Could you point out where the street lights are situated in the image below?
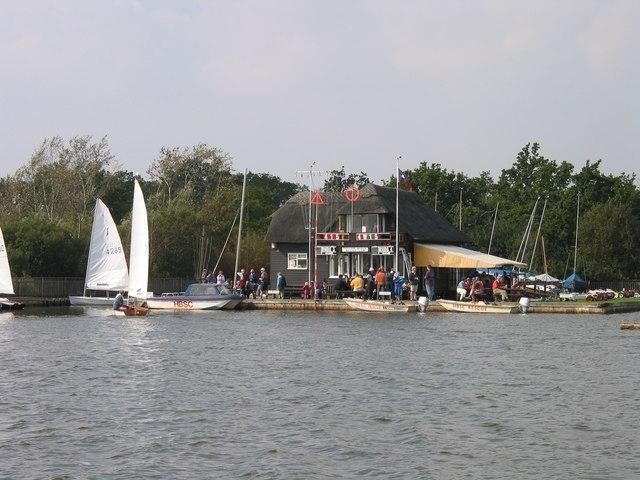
[394,155,402,277]
[294,161,331,298]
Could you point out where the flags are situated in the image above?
[398,170,413,193]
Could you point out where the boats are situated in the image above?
[132,282,244,311]
[437,295,530,314]
[342,296,429,313]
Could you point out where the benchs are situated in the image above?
[336,290,358,300]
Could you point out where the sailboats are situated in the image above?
[113,178,151,316]
[69,198,153,308]
[0,226,25,312]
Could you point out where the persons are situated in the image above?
[408,265,420,301]
[456,270,521,302]
[276,271,287,300]
[332,265,406,301]
[200,267,271,300]
[423,264,437,301]
